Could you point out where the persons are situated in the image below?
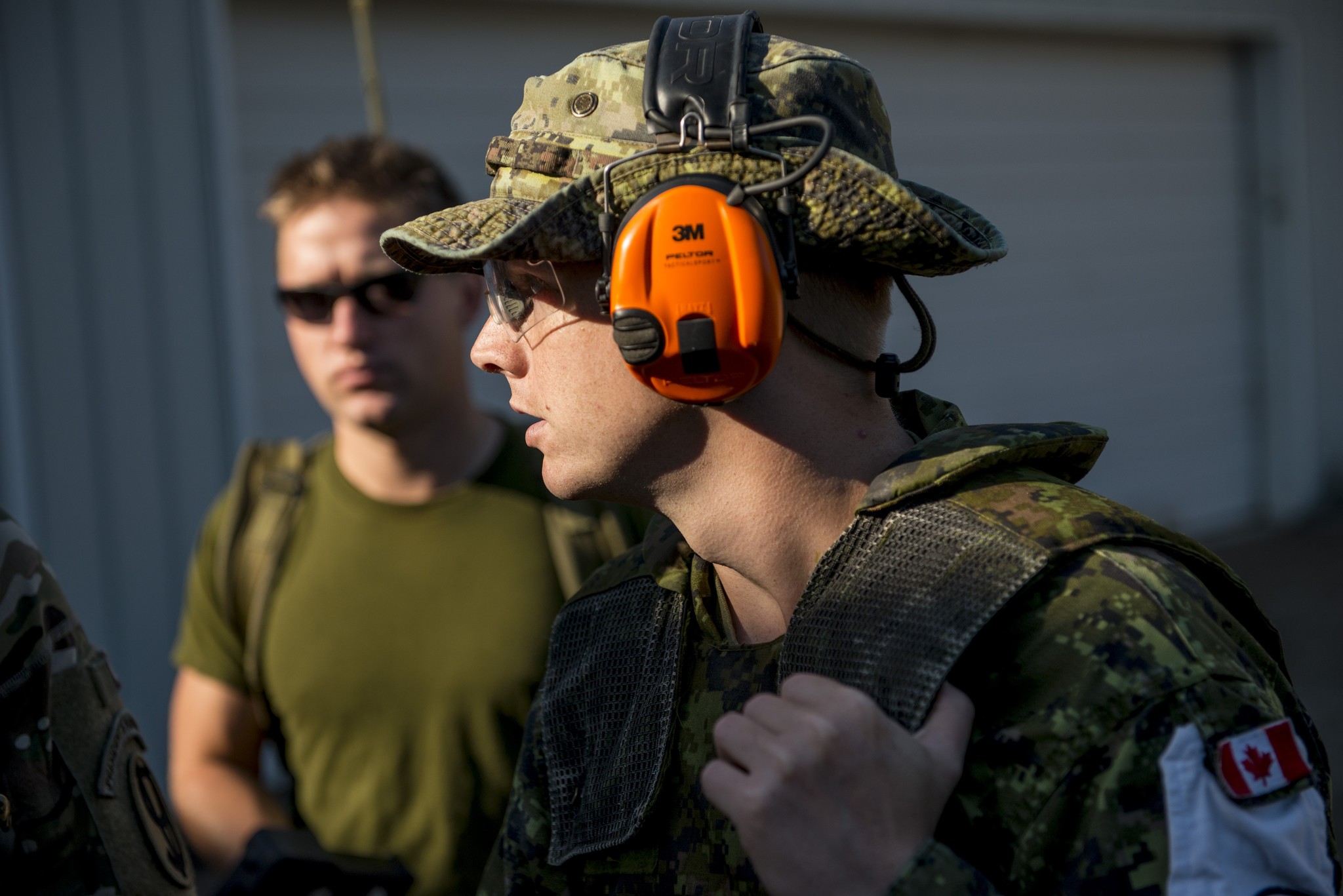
[0,504,205,895]
[159,132,636,896]
[375,16,1343,895]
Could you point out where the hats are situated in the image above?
[381,31,1007,278]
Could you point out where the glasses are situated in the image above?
[482,257,544,333]
[276,268,426,326]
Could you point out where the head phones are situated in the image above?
[598,9,784,411]
